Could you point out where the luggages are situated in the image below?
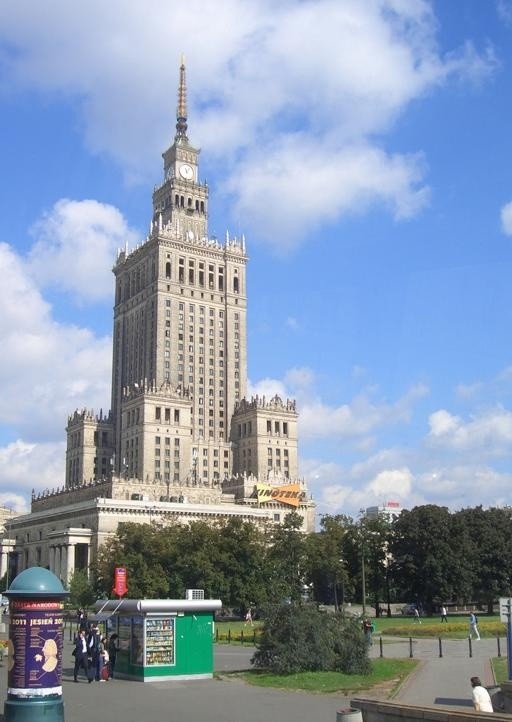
[101,664,109,681]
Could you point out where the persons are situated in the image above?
[470,675,494,713]
[413,608,422,624]
[360,617,376,649]
[243,608,255,626]
[440,605,448,624]
[70,607,121,684]
[466,611,481,641]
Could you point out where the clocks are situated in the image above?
[165,164,193,180]
